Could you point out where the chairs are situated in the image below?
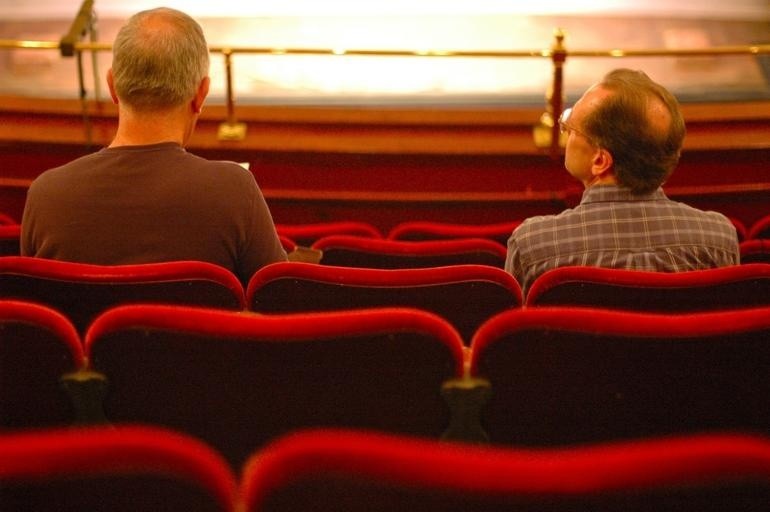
[467,304,769,424]
[2,422,236,512]
[246,261,524,345]
[524,261,770,306]
[1,254,246,339]
[237,424,769,512]
[2,177,748,261]
[86,302,467,424]
[0,299,85,421]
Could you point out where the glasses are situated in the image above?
[558,107,599,147]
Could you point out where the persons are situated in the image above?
[19,6,290,290]
[504,66,741,304]
